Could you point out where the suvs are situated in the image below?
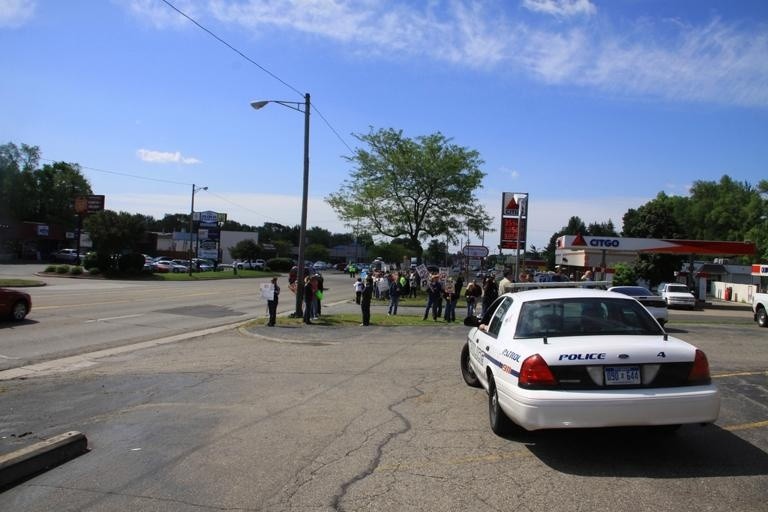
[236,258,265,270]
[695,262,731,276]
[659,283,696,309]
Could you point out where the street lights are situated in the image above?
[189,182,211,278]
[510,193,530,285]
[248,89,309,316]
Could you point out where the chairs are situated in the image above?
[531,306,627,330]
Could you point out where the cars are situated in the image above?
[0,286,33,323]
[137,254,217,273]
[657,281,666,296]
[456,286,721,441]
[305,260,370,272]
[598,284,667,328]
[452,265,498,278]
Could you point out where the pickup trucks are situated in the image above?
[750,291,768,328]
[55,247,86,258]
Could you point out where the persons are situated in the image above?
[289,260,323,325]
[267,276,280,327]
[233,259,237,275]
[346,256,596,326]
